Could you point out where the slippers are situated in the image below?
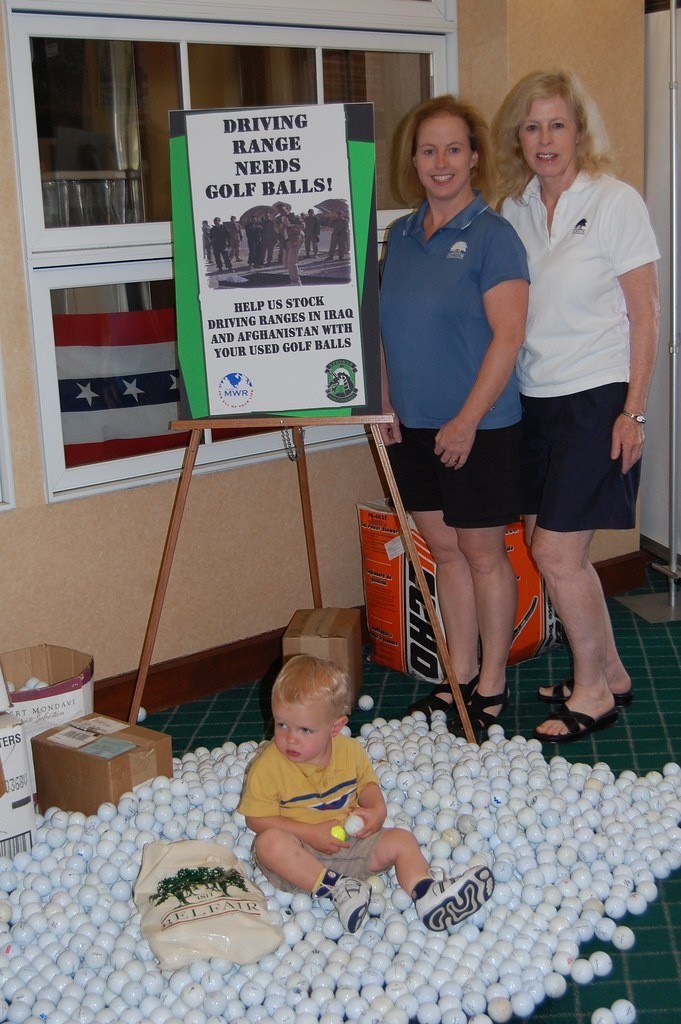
[532,698,619,743]
[538,676,635,708]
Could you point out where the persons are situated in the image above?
[377,95,534,746]
[490,69,661,744]
[199,199,350,287]
[235,658,497,934]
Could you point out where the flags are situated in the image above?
[54,308,295,470]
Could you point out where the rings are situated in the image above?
[448,458,458,464]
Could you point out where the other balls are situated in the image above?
[1,692,681,1023]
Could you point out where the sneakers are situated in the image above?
[416,866,495,930]
[313,872,371,934]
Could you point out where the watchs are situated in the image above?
[622,410,646,424]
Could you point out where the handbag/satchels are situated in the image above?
[132,839,284,969]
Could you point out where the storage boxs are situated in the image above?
[0,643,173,859]
[282,607,365,705]
[357,497,566,683]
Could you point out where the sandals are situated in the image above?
[407,673,481,724]
[450,679,511,734]
